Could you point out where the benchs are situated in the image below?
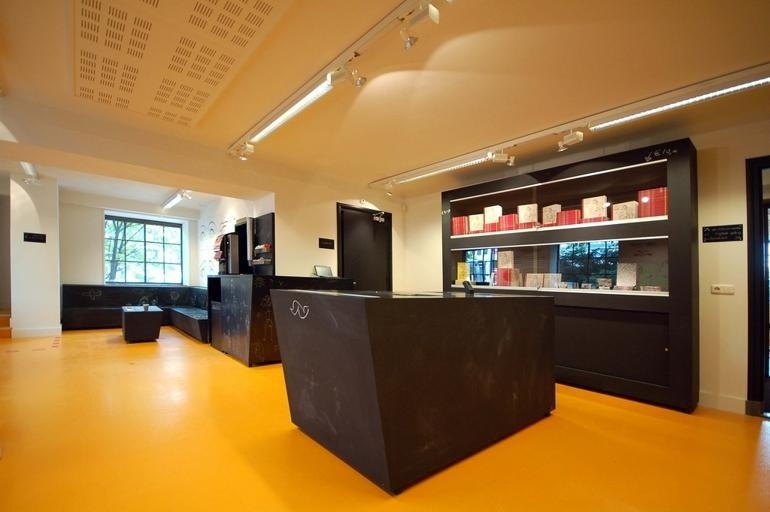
[62,284,211,346]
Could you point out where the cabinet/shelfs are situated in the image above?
[439,152,701,415]
